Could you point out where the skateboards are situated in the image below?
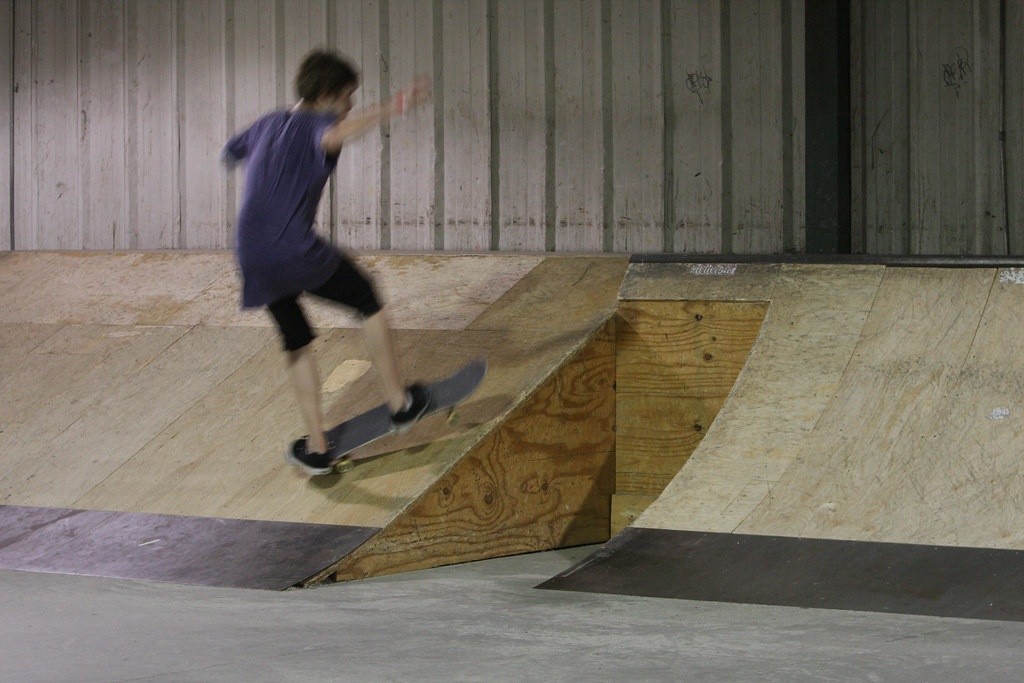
[292,355,488,473]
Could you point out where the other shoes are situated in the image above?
[390,382,432,434]
[291,436,332,474]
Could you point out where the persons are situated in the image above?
[222,49,432,475]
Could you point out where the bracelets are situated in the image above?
[395,92,403,113]
